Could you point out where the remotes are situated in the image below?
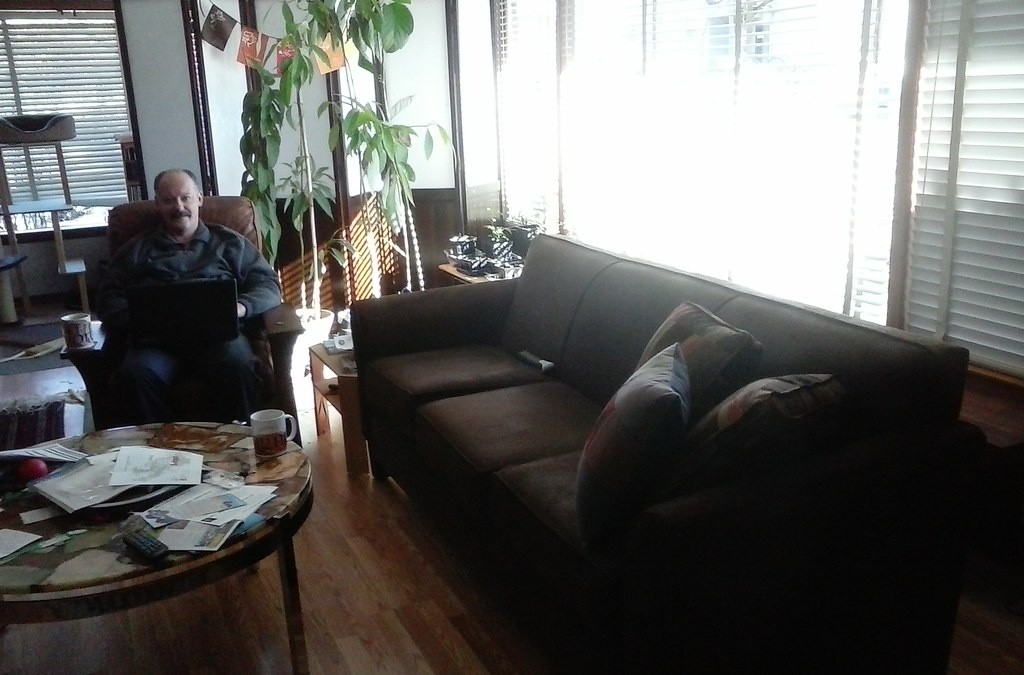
[123,529,167,560]
[517,350,555,373]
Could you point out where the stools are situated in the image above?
[0,255,29,326]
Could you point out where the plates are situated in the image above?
[90,446,173,509]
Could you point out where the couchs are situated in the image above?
[349,233,973,675]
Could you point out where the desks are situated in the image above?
[0,205,113,247]
[308,341,371,476]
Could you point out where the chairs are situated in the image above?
[58,192,305,457]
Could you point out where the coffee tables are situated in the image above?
[0,422,315,675]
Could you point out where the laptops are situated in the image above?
[128,278,239,345]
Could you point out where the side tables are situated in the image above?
[436,258,490,286]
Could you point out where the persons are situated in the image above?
[95,168,284,425]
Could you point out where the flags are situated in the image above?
[314,34,345,75]
[237,26,269,70]
[277,38,295,75]
[201,5,237,51]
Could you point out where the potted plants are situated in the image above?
[240,1,456,352]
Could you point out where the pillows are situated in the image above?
[689,370,849,491]
[573,336,693,517]
[624,297,767,424]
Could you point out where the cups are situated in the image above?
[250,409,297,458]
[61,313,95,350]
